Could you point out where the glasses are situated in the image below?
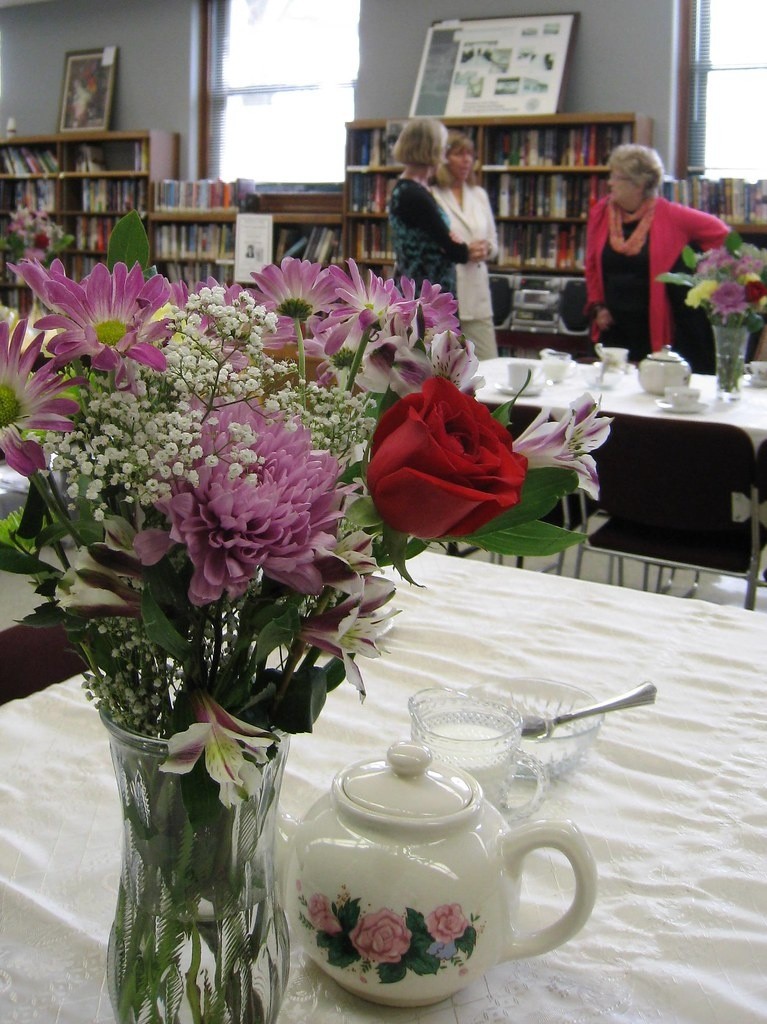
[608,172,634,183]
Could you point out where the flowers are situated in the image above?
[655,231,767,330]
[0,208,73,269]
[0,210,614,778]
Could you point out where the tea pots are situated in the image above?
[638,344,692,394]
[287,742,597,1008]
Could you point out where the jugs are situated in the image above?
[539,348,576,383]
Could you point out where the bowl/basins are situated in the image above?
[466,677,604,780]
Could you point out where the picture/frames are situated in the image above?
[57,47,119,135]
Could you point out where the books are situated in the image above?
[349,220,400,260]
[486,173,611,219]
[348,174,399,214]
[156,224,232,260]
[0,142,147,319]
[274,227,344,264]
[485,123,634,164]
[490,221,591,268]
[162,264,235,296]
[652,176,767,225]
[350,127,476,164]
[152,178,255,211]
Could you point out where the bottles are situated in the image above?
[593,342,628,389]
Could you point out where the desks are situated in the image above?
[470,359,767,453]
[0,550,767,1024]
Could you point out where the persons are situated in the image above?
[388,116,499,361]
[584,144,731,375]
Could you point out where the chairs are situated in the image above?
[447,356,598,576]
[573,410,767,612]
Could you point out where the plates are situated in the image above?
[749,378,767,387]
[656,400,708,412]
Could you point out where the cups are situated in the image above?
[663,386,700,402]
[743,359,767,379]
[506,359,540,390]
[409,686,550,822]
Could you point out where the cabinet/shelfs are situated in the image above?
[0,112,767,342]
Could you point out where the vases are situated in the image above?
[100,712,289,1024]
[711,325,749,400]
